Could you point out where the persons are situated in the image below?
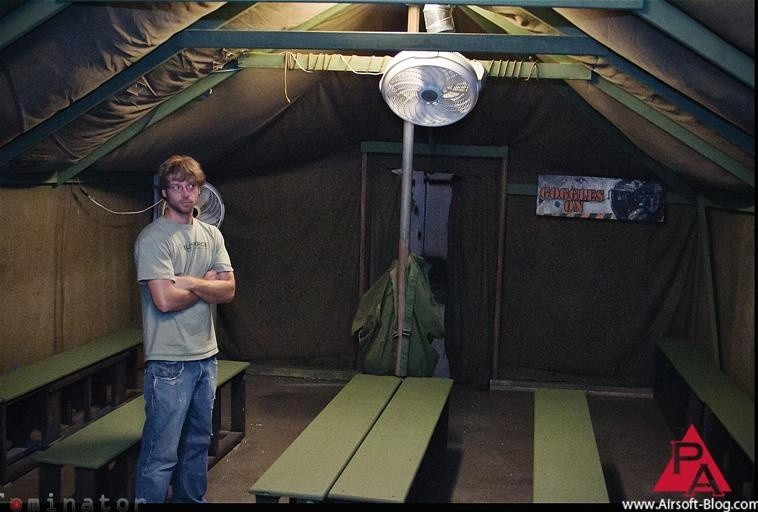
[133,155,235,512]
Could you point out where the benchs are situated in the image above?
[657,334,755,465]
[533,386,611,503]
[1,326,146,483]
[249,373,401,505]
[32,360,250,507]
[326,377,454,504]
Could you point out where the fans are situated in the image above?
[377,51,484,128]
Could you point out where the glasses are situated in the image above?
[165,182,199,192]
